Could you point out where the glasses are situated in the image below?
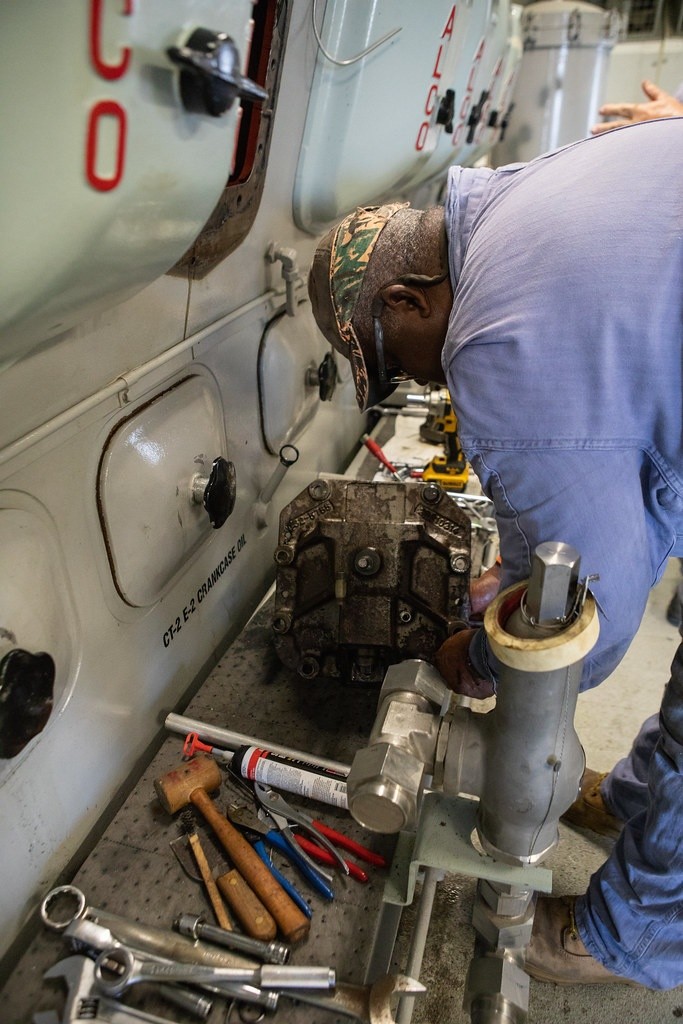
[371,273,446,385]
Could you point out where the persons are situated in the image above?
[588,79,683,135]
[305,116,683,993]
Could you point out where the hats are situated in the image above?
[307,199,410,414]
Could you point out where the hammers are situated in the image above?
[152,753,311,945]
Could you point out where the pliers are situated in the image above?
[255,782,385,883]
[226,802,336,920]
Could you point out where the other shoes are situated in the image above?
[525,893,652,991]
[560,765,626,839]
